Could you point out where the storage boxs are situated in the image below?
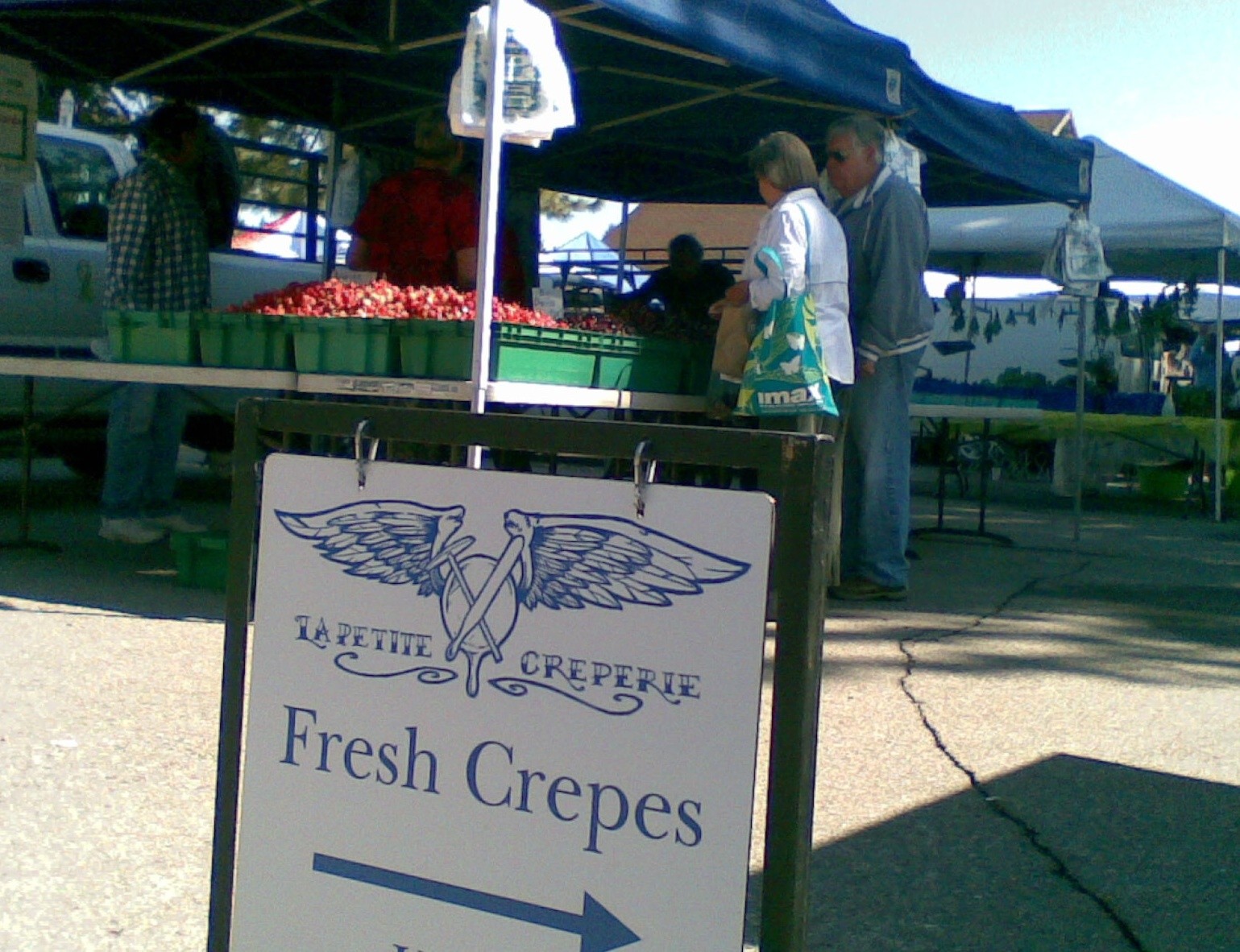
[108,311,691,590]
[912,378,1173,418]
[0,56,39,188]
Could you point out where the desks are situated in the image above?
[908,405,1240,548]
[295,373,713,495]
[0,352,300,553]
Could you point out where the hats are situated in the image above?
[149,102,214,138]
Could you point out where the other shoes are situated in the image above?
[828,576,910,600]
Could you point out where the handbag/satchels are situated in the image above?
[712,203,840,419]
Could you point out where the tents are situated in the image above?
[0,0,1240,551]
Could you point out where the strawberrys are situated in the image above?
[213,278,640,334]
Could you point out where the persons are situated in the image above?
[345,107,525,463]
[98,105,214,546]
[1189,324,1230,490]
[708,131,855,587]
[617,234,735,323]
[131,99,243,250]
[827,114,935,603]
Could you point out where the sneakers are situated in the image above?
[96,517,166,545]
[144,514,209,533]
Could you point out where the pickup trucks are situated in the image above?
[0,122,385,486]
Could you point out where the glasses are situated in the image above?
[824,147,865,163]
